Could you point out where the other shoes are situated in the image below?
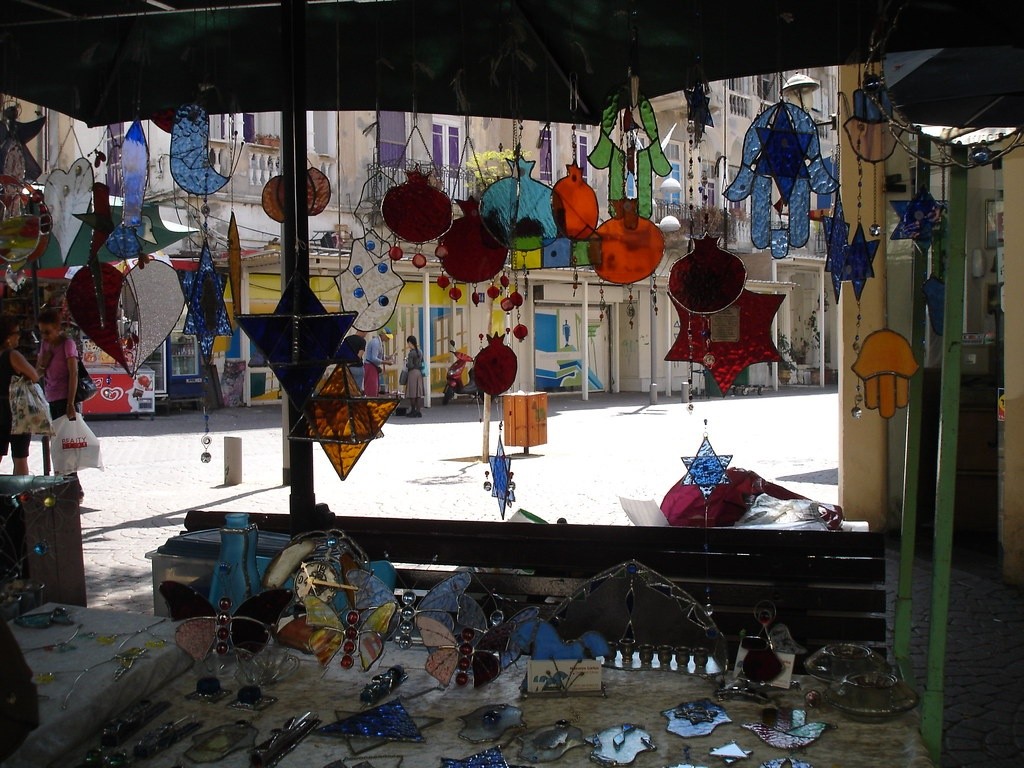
[407,410,423,418]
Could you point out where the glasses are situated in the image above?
[39,329,57,335]
[11,330,23,337]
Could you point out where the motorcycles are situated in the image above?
[442,339,496,405]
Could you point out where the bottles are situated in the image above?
[171,333,195,375]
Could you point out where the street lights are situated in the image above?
[649,174,681,405]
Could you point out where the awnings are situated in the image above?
[0,0,1024,128]
[219,248,800,292]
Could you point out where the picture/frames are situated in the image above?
[985,199,1004,249]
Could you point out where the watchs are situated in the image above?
[67,403,74,407]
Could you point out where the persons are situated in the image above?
[363,327,394,397]
[340,330,366,388]
[35,309,85,504]
[405,336,425,418]
[0,316,40,475]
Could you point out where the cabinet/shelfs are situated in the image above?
[954,406,998,532]
[0,472,87,608]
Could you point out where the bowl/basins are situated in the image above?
[803,644,891,686]
[823,671,919,722]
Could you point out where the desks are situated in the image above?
[5,602,934,768]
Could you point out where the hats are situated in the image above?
[381,327,393,339]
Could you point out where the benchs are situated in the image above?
[158,509,888,657]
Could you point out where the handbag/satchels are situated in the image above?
[377,367,382,374]
[8,375,57,436]
[68,360,97,405]
[51,412,105,474]
[398,370,408,385]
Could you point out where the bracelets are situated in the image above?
[39,364,45,369]
